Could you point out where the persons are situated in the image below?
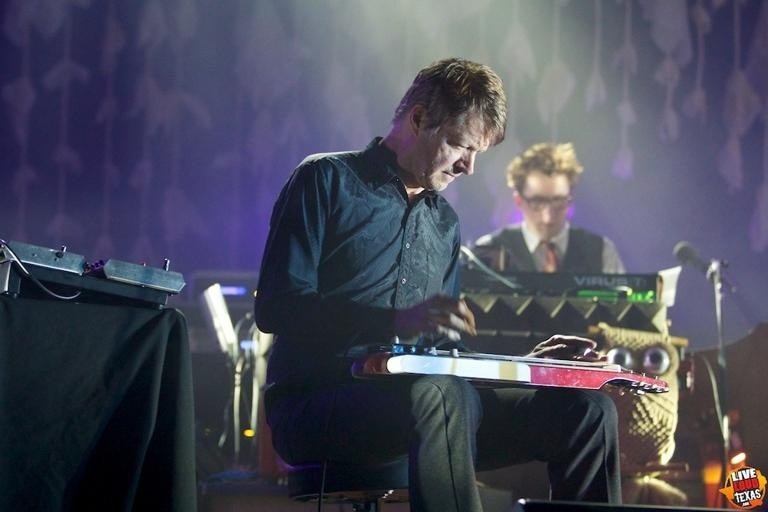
[467,138,629,320]
[255,58,670,510]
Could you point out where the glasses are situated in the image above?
[520,190,577,212]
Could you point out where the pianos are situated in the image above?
[181,267,681,307]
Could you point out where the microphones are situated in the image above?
[672,241,737,294]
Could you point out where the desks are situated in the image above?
[1,293,196,512]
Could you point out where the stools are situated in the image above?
[286,458,408,512]
